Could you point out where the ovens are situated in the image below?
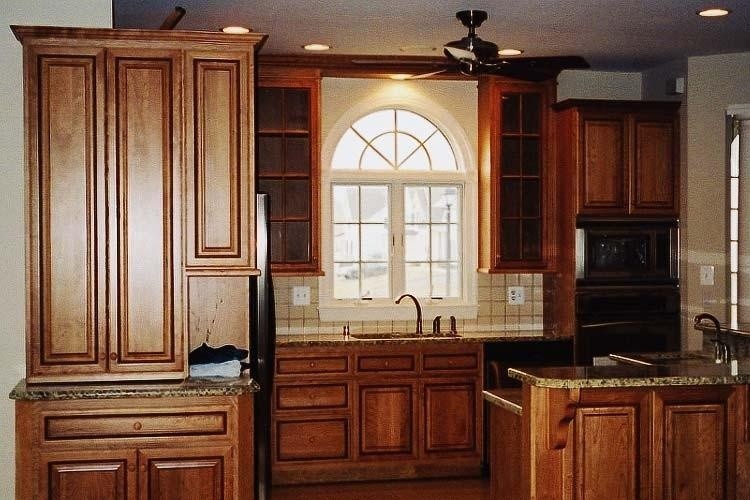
[576,291,681,366]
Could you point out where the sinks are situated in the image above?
[422,333,462,337]
[352,330,420,338]
[640,351,706,362]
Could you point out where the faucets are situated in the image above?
[394,293,423,334]
[693,313,723,366]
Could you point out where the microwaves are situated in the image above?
[577,226,674,281]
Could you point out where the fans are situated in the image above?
[408,13,589,86]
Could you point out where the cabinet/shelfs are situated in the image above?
[185,50,252,268]
[23,45,186,374]
[276,354,350,462]
[421,352,480,452]
[36,404,235,500]
[492,80,558,274]
[578,107,681,217]
[255,67,321,270]
[356,354,417,456]
